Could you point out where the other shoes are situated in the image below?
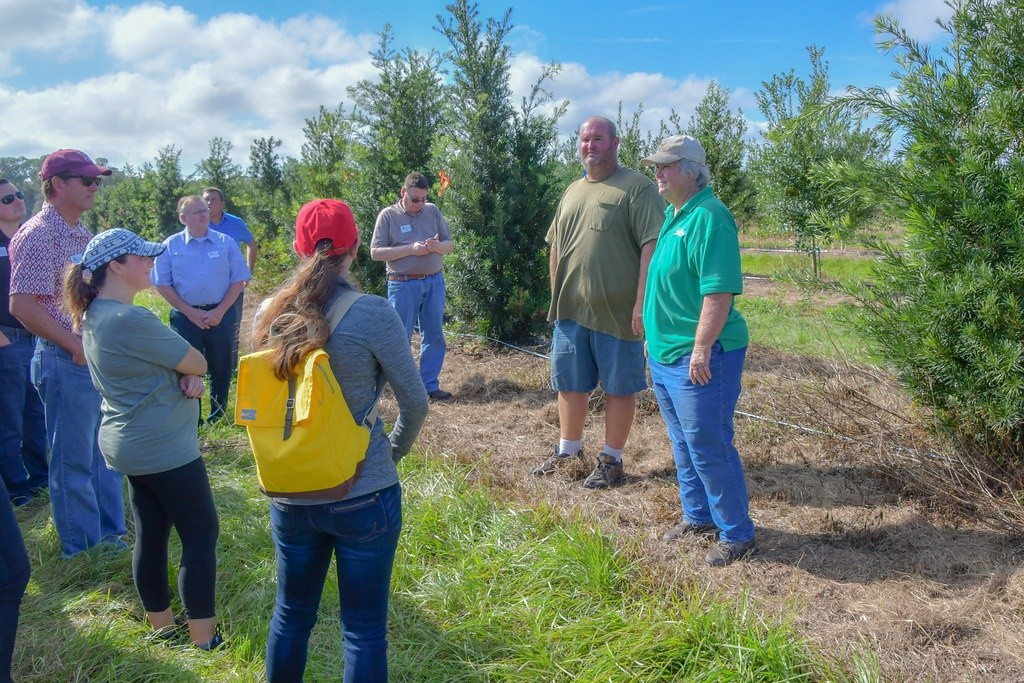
[206,622,228,651]
[426,389,453,400]
[9,492,36,511]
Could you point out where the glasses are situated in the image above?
[0,191,25,206]
[62,174,102,187]
[183,207,211,219]
[404,189,427,203]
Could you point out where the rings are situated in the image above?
[201,382,205,388]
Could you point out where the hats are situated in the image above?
[296,200,359,260]
[639,135,707,170]
[41,148,112,182]
[81,227,167,272]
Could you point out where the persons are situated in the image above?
[641,135,759,565]
[7,149,130,558]
[232,198,429,683]
[0,179,49,505]
[63,228,232,652]
[150,195,253,426]
[371,172,453,401]
[201,187,258,383]
[0,477,32,682]
[531,115,668,489]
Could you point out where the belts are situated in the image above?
[388,273,432,282]
[193,304,217,312]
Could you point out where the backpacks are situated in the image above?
[234,291,391,499]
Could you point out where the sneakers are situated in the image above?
[529,443,585,477]
[661,520,717,545]
[705,536,756,566]
[583,453,624,489]
